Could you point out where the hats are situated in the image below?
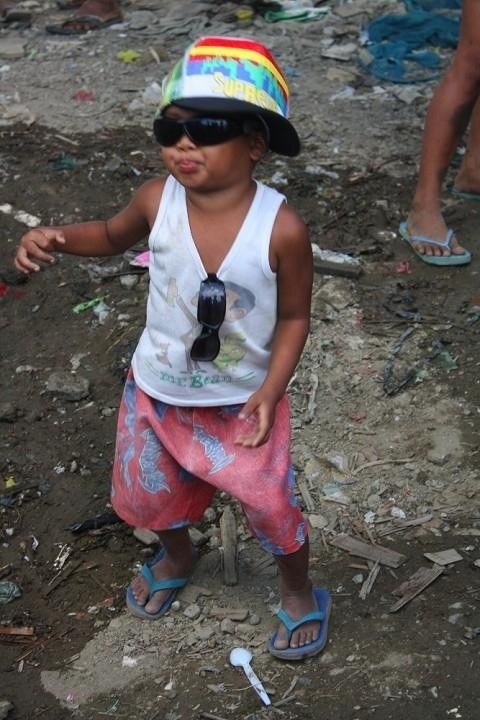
[155,35,300,156]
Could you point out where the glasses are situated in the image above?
[190,273,226,362]
[154,114,246,146]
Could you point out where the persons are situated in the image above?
[46,0,123,35]
[13,35,334,660]
[398,0,480,266]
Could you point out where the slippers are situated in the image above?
[399,222,471,266]
[125,545,191,621]
[267,586,332,660]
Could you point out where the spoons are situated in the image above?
[229,646,272,707]
